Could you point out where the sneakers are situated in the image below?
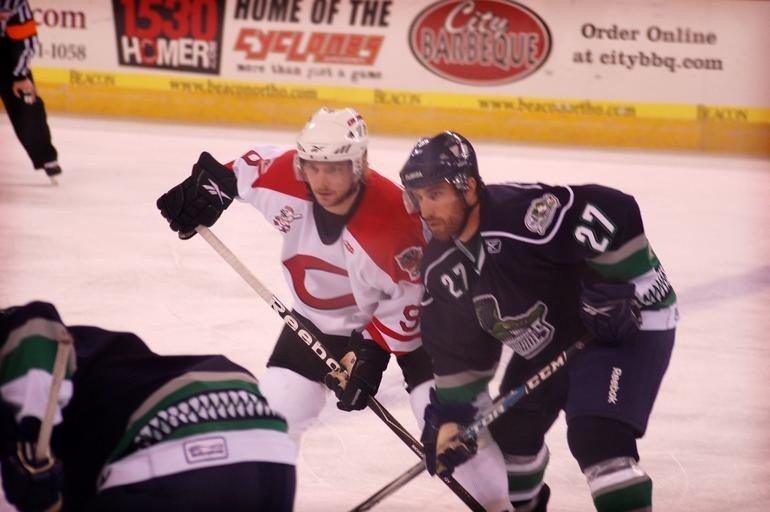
[43,162,61,175]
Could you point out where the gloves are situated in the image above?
[324,329,389,410]
[579,279,644,345]
[156,152,237,240]
[0,441,67,512]
[421,387,478,477]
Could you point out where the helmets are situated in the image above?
[399,130,479,211]
[294,108,369,181]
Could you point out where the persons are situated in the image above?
[399,130,679,512]
[0,0,62,177]
[0,300,296,512]
[156,107,514,512]
